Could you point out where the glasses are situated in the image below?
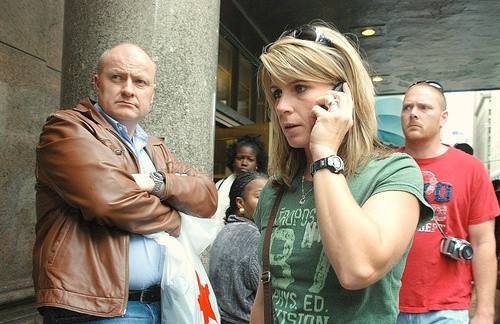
[409,79,443,90]
[278,26,335,48]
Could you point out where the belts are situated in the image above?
[127,287,160,304]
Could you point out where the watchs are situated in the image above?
[307,152,345,178]
[148,171,167,195]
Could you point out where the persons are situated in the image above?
[450,142,475,157]
[207,133,264,221]
[32,41,219,323]
[490,178,499,291]
[247,19,424,323]
[207,168,270,323]
[381,78,500,323]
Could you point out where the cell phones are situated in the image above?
[320,80,343,111]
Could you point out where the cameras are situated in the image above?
[441,236,474,260]
